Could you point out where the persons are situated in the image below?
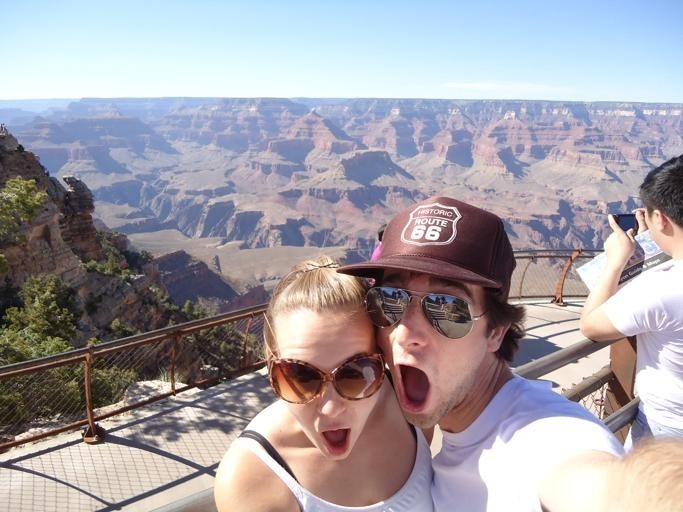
[214,257,434,512]
[604,435,683,512]
[578,155,683,458]
[367,224,386,285]
[336,194,624,512]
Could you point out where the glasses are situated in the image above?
[266,351,387,403]
[363,285,486,340]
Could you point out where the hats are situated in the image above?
[337,194,516,289]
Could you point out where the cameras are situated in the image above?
[615,213,640,235]
